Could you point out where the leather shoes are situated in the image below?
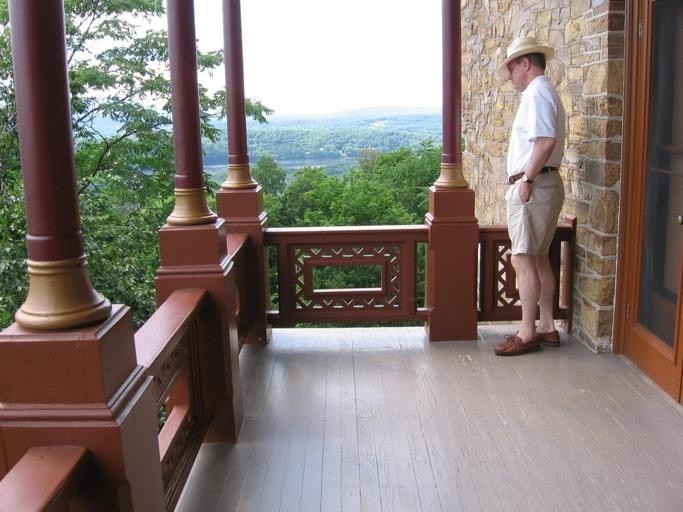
[493,333,541,357]
[535,329,561,347]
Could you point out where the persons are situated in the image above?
[495,36,566,356]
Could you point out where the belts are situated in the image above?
[508,164,558,186]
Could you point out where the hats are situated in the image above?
[496,36,556,80]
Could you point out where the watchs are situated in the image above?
[521,174,533,184]
